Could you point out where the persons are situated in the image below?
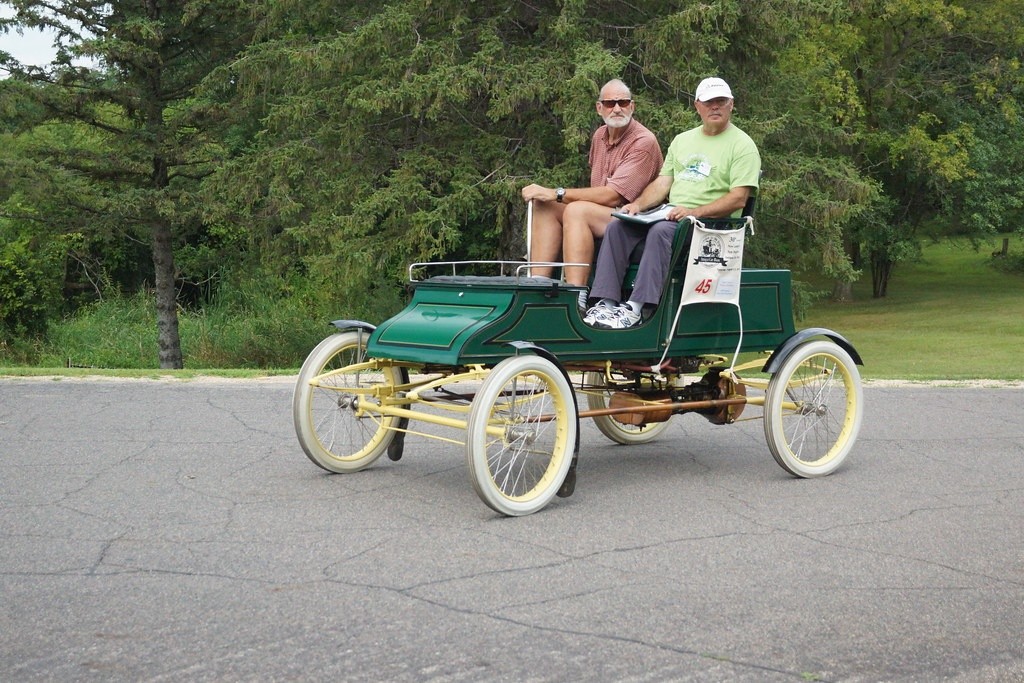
[583,76,761,332]
[522,77,664,322]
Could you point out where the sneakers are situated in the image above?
[583,298,614,327]
[593,302,643,329]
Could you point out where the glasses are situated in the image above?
[696,99,730,107]
[598,99,631,109]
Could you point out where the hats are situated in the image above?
[695,77,734,102]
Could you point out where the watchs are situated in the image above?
[556,187,566,204]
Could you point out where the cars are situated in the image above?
[293,171,866,519]
